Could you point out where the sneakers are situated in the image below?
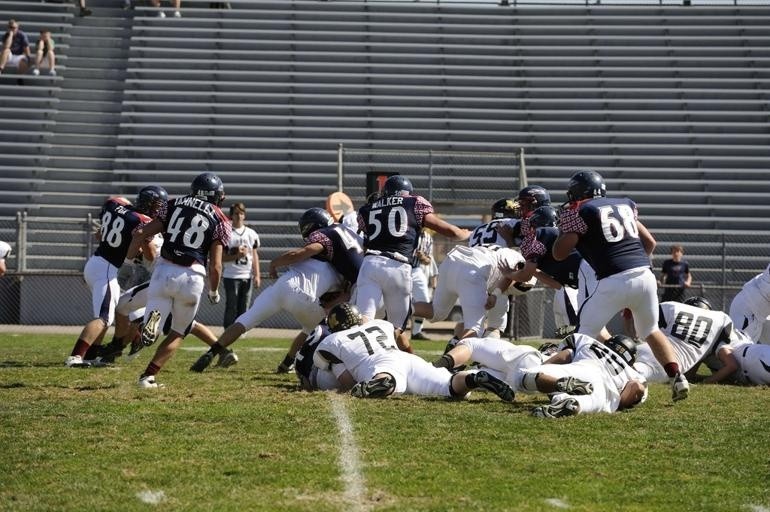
[216,351,240,368]
[533,398,580,419]
[474,371,515,401]
[190,352,214,373]
[68,341,124,368]
[128,310,162,389]
[556,377,594,396]
[672,373,690,402]
[278,363,296,373]
[351,377,396,399]
[412,332,431,341]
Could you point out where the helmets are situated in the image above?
[138,186,170,218]
[192,174,226,206]
[300,207,335,239]
[684,296,713,311]
[328,303,364,334]
[367,192,384,205]
[384,176,414,197]
[492,169,609,233]
[604,334,638,367]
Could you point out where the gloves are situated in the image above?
[209,290,221,306]
[117,258,137,292]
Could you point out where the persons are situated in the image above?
[62,168,770,422]
[32,27,57,76]
[79,0,93,18]
[0,239,12,276]
[0,18,32,86]
[152,0,182,18]
